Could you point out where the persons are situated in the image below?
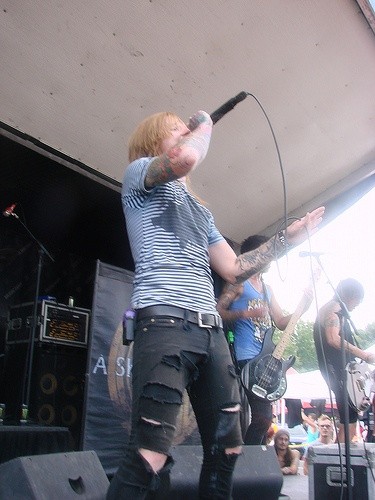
[273,429,300,475]
[216,234,314,444]
[313,278,375,444]
[300,407,319,451]
[106,109,325,500]
[302,413,336,475]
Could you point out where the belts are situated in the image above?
[137,306,223,329]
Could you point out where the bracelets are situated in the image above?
[278,231,292,249]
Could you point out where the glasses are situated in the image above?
[319,424,331,429]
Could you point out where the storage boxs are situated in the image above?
[7,297,91,350]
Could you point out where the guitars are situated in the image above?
[240,268,322,403]
[346,360,375,414]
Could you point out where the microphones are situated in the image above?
[3,200,18,216]
[210,90,248,125]
[299,250,325,257]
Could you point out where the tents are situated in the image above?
[281,369,337,427]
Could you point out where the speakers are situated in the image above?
[146,444,284,500]
[0,450,111,500]
[2,342,89,451]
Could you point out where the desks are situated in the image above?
[0,426,70,463]
